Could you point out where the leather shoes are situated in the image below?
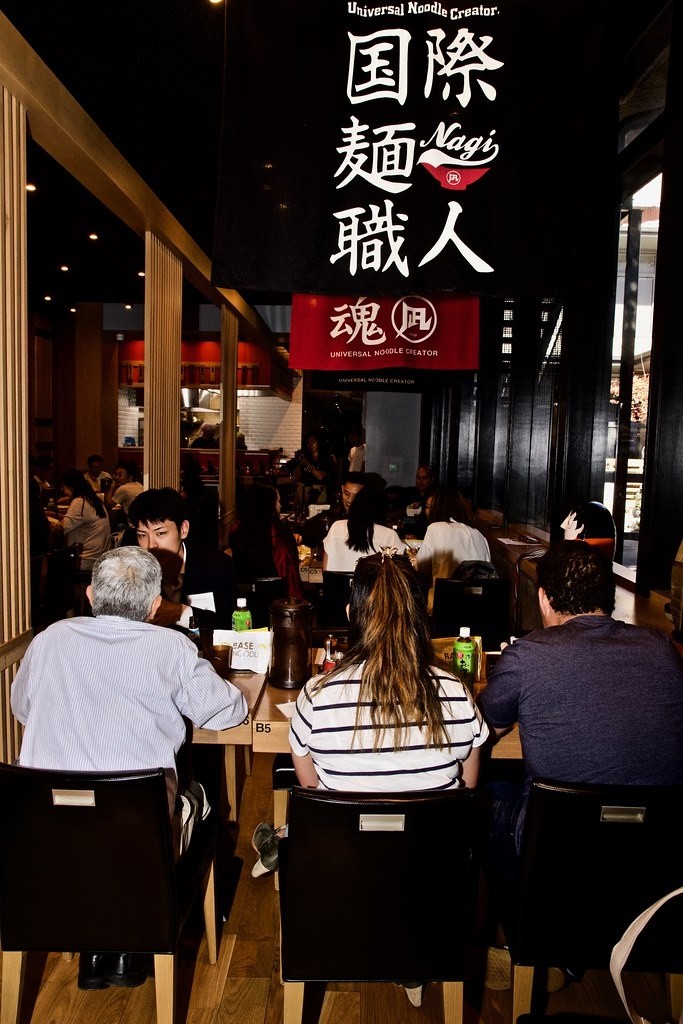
[77,952,110,990]
[103,952,149,987]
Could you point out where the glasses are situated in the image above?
[112,471,125,478]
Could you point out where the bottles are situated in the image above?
[323,633,337,660]
[232,598,253,631]
[186,616,204,658]
[453,627,476,694]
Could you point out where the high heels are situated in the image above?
[251,822,282,878]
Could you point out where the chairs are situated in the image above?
[220,571,286,634]
[431,577,513,648]
[277,792,492,1023]
[0,763,217,1024]
[505,782,682,1023]
[32,541,81,629]
[319,571,359,626]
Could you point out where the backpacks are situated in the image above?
[451,559,500,587]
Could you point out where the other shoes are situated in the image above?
[484,947,566,994]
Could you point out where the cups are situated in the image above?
[202,645,234,677]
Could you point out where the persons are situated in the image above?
[231,482,302,622]
[473,539,683,975]
[190,423,249,449]
[10,547,248,990]
[28,451,218,618]
[126,491,260,637]
[282,427,365,507]
[396,466,492,617]
[296,469,407,638]
[252,549,491,1007]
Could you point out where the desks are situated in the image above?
[41,503,550,823]
